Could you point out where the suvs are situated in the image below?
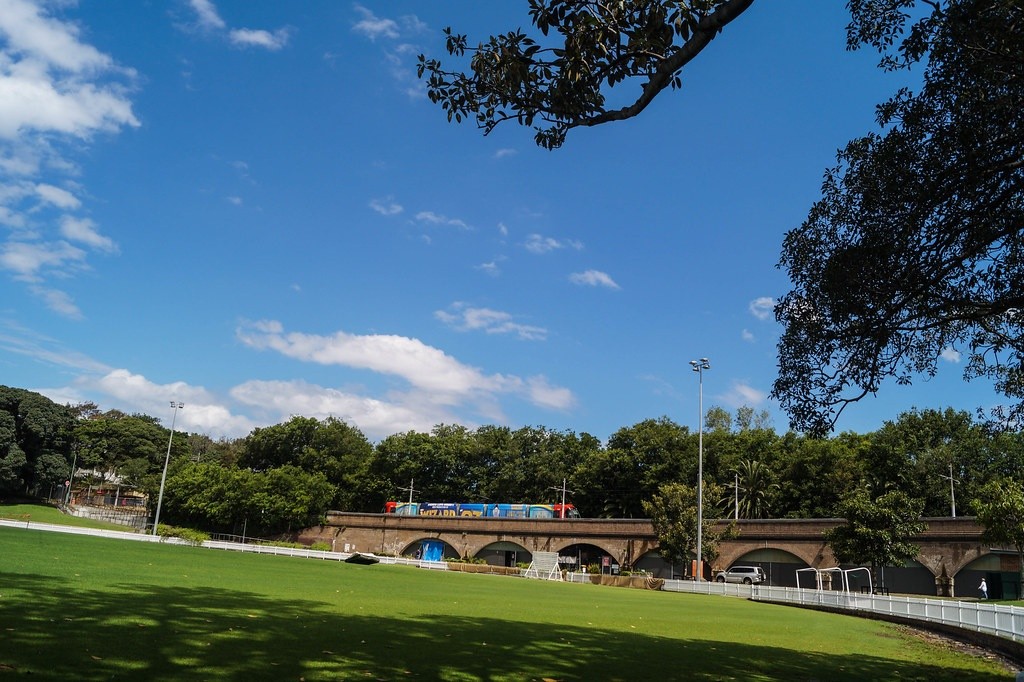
[716,566,767,585]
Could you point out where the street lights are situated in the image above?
[688,356,711,582]
[152,401,185,535]
[396,477,421,514]
[937,463,960,518]
[723,473,747,519]
[545,478,576,519]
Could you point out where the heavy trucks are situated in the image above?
[380,501,582,518]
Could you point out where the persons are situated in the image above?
[978,578,988,599]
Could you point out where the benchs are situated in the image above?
[673,575,695,580]
[861,586,889,596]
